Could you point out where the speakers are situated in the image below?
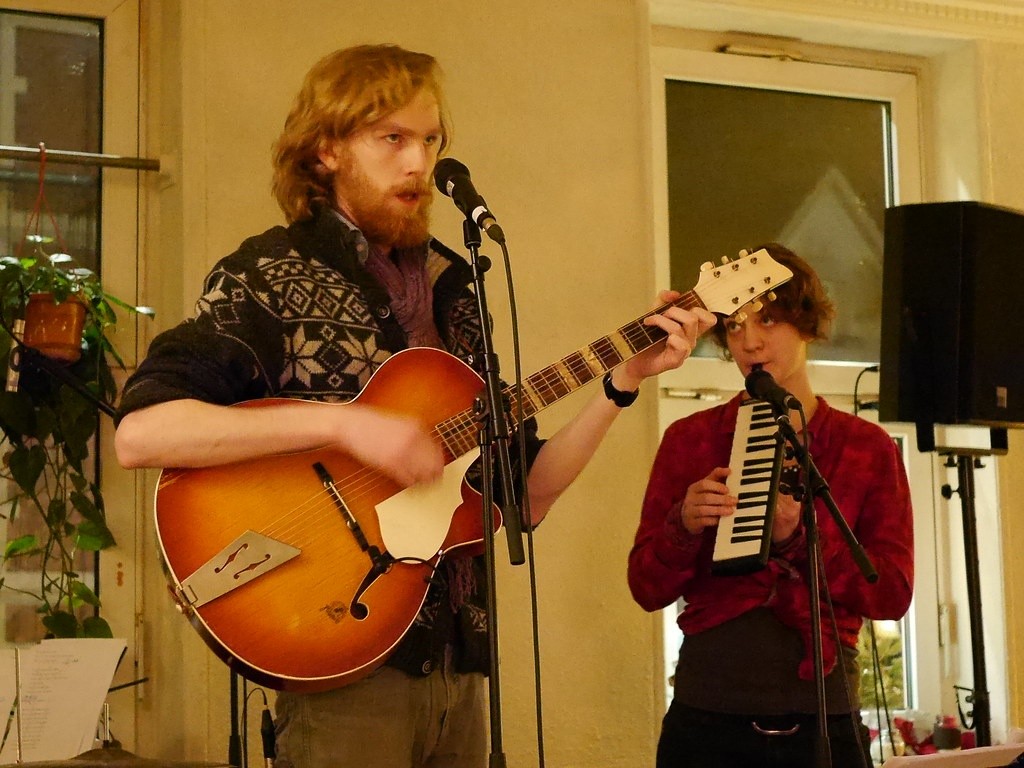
[878,201,1024,429]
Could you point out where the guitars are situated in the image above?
[154,249,792,695]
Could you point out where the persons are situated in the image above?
[114,41,716,768]
[626,243,915,768]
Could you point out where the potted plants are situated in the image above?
[0,253,157,639]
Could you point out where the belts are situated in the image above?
[746,709,805,736]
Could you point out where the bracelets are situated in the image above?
[603,371,639,407]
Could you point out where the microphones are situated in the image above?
[434,158,506,245]
[744,370,802,410]
